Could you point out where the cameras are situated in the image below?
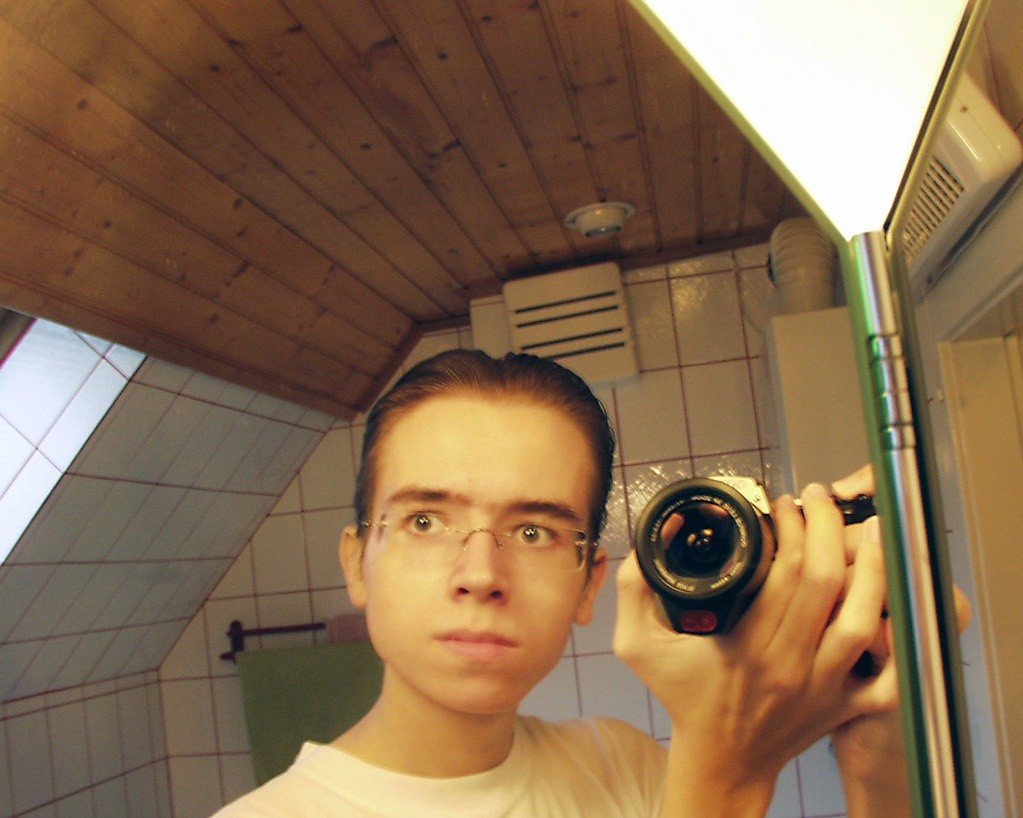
[636,474,887,638]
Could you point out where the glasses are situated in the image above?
[361,510,598,572]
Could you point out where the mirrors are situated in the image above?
[0,0,944,818]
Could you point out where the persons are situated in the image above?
[210,349,972,818]
